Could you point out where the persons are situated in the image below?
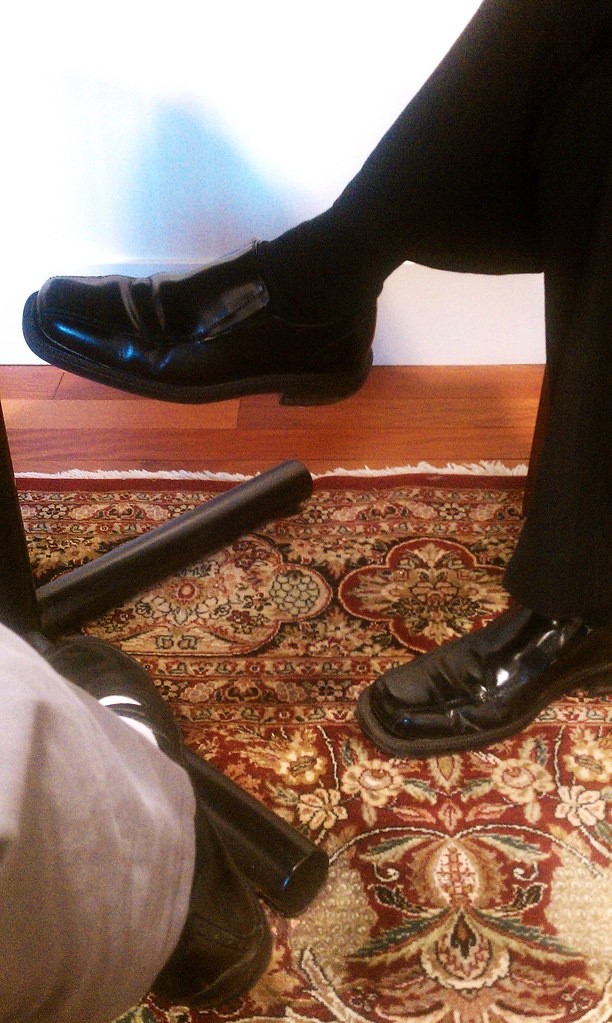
[0,623,271,1023]
[20,0,612,758]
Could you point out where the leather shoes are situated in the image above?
[40,635,272,1011]
[20,240,378,409]
[355,605,612,759]
